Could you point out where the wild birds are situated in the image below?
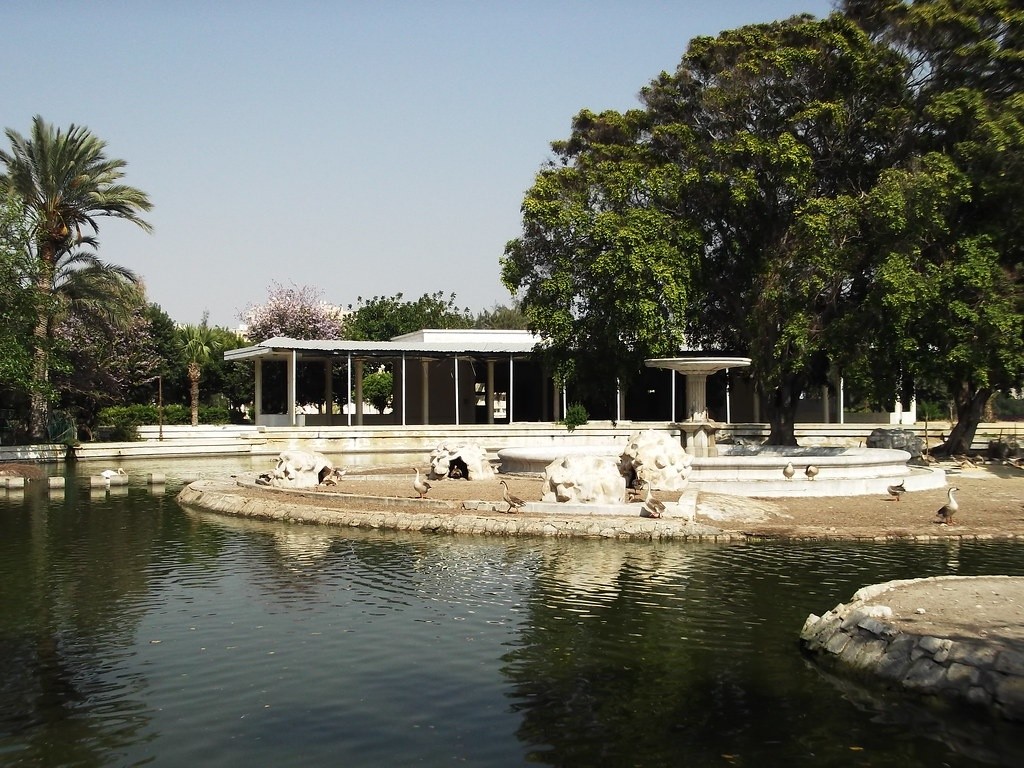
[935,487,960,525]
[888,478,907,502]
[804,464,822,480]
[641,479,667,519]
[498,480,527,514]
[100,468,127,477]
[450,464,463,478]
[413,467,432,500]
[782,461,796,480]
